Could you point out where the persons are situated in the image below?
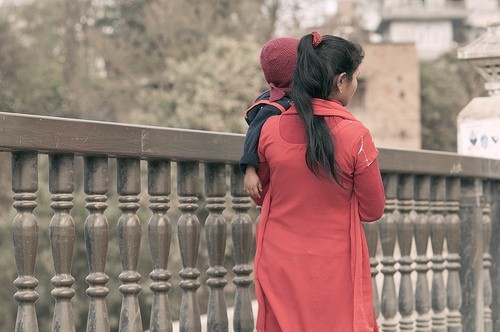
[240,37,302,198]
[253,32,386,332]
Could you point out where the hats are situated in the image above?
[260,37,300,102]
